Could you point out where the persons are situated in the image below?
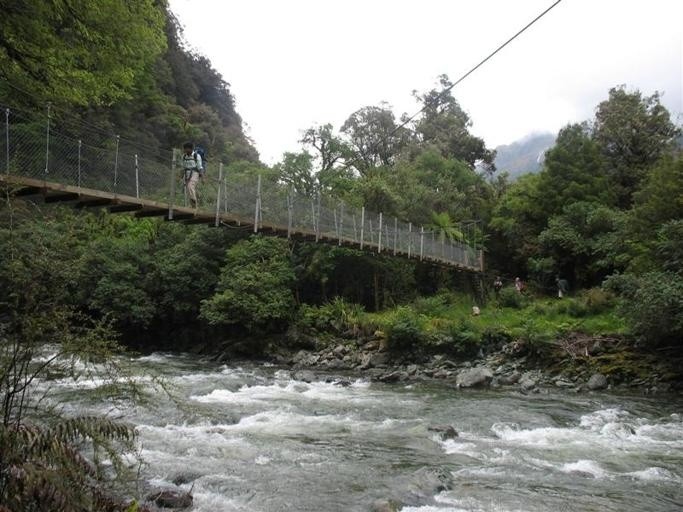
[494,277,503,299]
[179,141,205,212]
[515,277,524,294]
[554,278,566,300]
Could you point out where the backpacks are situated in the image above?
[183,147,207,177]
